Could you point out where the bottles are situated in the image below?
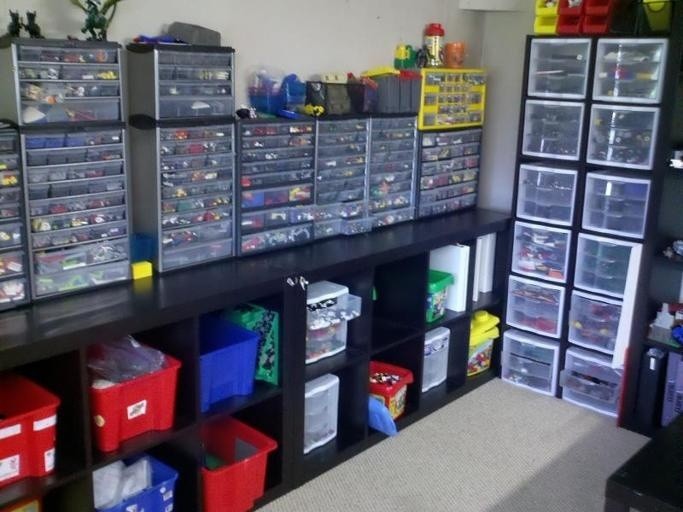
[425,24,444,68]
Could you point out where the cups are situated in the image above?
[440,41,465,68]
[393,44,424,70]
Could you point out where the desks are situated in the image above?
[604,412,683,512]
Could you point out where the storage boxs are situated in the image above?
[305,280,362,365]
[0,375,60,487]
[198,415,278,512]
[99,455,178,512]
[304,373,340,453]
[422,326,450,392]
[88,344,181,451]
[197,315,261,413]
[368,361,413,422]
[425,268,455,323]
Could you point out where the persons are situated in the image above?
[416,43,436,69]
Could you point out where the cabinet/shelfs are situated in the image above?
[618,0,683,440]
[1,21,487,311]
[243,207,514,489]
[498,36,672,419]
[0,258,299,512]
